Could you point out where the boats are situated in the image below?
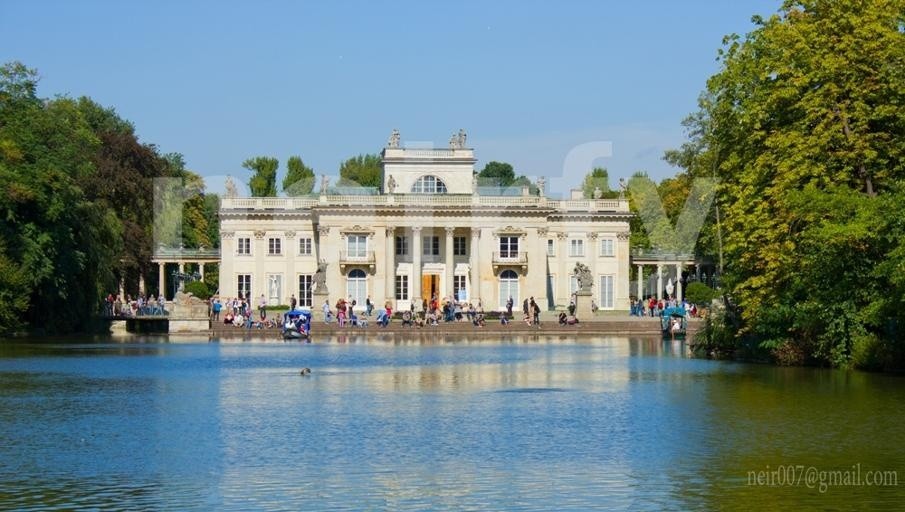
[659,307,687,339]
[282,310,312,339]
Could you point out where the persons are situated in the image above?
[536,176,544,196]
[387,175,396,193]
[449,133,457,149]
[630,295,697,341]
[387,129,400,148]
[224,173,234,198]
[205,293,597,336]
[103,293,165,318]
[593,187,603,199]
[618,177,626,199]
[312,259,329,292]
[574,261,593,291]
[321,173,329,195]
[458,128,465,148]
